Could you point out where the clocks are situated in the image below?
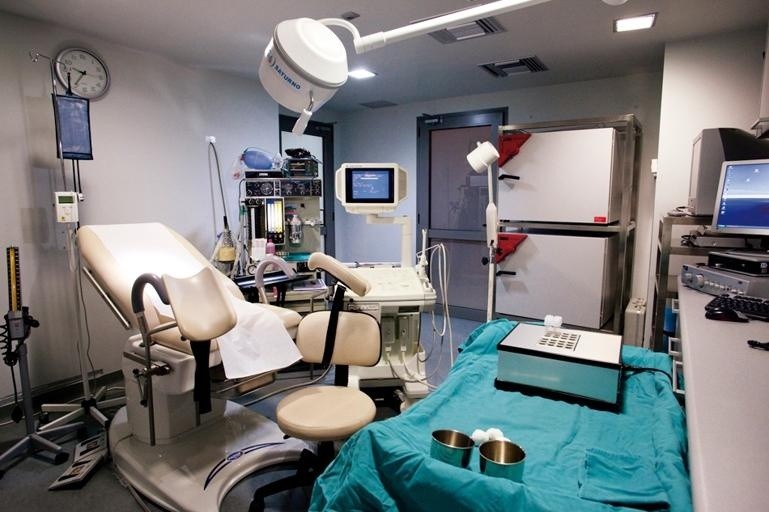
[55,47,109,100]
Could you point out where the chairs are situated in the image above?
[248,310,381,512]
[73,221,371,512]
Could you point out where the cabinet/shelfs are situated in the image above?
[493,113,643,334]
[650,215,769,352]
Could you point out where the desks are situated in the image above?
[675,275,769,511]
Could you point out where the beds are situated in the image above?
[306,318,693,512]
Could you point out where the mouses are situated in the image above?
[708,305,750,323]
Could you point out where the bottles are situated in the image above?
[290,214,303,244]
[267,239,275,271]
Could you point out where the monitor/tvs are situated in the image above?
[334,163,409,216]
[710,157,769,260]
[686,127,765,218]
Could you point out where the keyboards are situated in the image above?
[705,289,769,325]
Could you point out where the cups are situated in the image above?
[430,429,474,468]
[480,440,527,483]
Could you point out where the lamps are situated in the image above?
[258,0,546,136]
[465,140,501,323]
[208,142,237,262]
